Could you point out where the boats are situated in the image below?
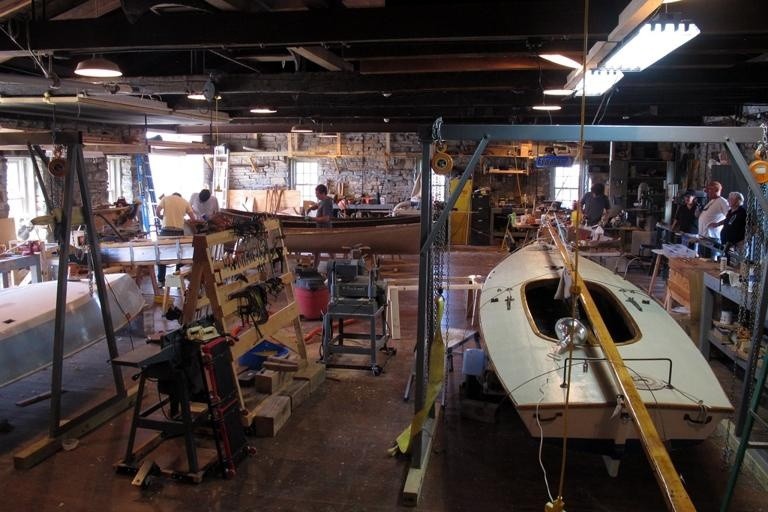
[1,274,146,389]
[476,238,734,443]
[217,207,428,256]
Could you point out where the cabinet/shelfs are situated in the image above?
[489,207,534,245]
[699,270,768,387]
[666,257,736,321]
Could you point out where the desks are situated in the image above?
[511,222,643,249]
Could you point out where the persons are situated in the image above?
[697,181,731,244]
[578,183,612,226]
[720,191,747,254]
[671,189,700,251]
[190,189,219,222]
[304,184,333,223]
[156,193,195,288]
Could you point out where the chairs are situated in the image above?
[613,245,662,280]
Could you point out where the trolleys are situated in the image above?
[313,258,396,380]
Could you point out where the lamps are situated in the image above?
[74,53,123,78]
